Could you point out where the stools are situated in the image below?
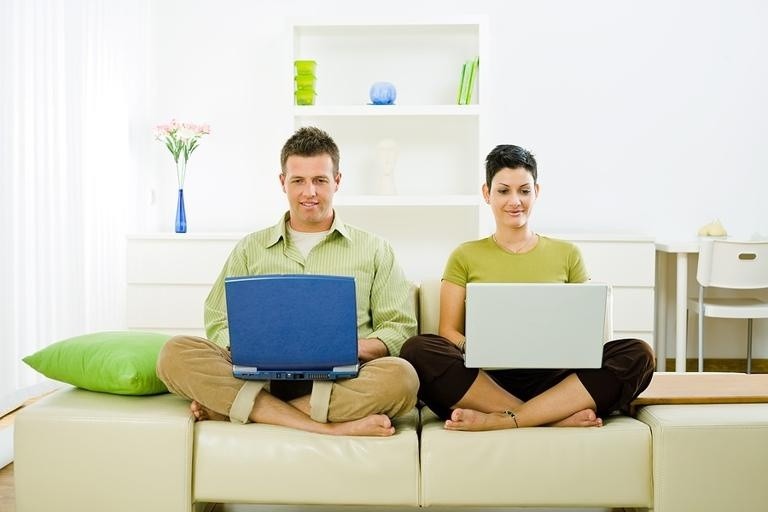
[12,388,218,512]
[634,401,768,511]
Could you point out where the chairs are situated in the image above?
[416,281,651,511]
[190,277,420,511]
[687,237,767,371]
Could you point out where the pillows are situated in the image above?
[23,328,168,397]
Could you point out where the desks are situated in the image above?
[655,231,767,372]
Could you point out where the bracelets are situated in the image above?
[454,336,467,353]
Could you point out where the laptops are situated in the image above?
[463,283,608,369]
[224,274,360,380]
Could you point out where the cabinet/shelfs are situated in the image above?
[117,227,259,343]
[554,234,657,372]
[289,20,489,240]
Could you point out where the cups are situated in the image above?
[369,83,396,106]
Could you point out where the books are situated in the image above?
[458,60,475,104]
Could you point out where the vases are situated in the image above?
[172,188,188,235]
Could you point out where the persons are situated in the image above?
[157,127,420,437]
[400,144,655,431]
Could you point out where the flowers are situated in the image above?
[152,116,211,227]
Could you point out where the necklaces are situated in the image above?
[494,231,535,254]
